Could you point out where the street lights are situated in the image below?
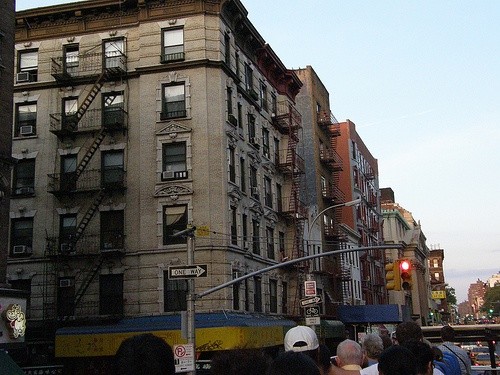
[309,198,362,363]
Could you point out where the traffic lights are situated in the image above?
[400,261,411,290]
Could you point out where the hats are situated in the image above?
[283,325,319,352]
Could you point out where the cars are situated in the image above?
[471,345,500,366]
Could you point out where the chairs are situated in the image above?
[433,351,462,375]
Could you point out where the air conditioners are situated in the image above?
[17,72,30,83]
[162,170,176,179]
[19,124,33,136]
[61,242,73,251]
[60,279,74,287]
[13,245,30,254]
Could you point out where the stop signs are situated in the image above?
[174,344,194,373]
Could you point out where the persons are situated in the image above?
[268,350,322,375]
[378,339,435,375]
[319,344,332,375]
[362,333,384,367]
[336,338,364,370]
[379,334,392,349]
[431,326,472,375]
[330,321,444,375]
[114,333,176,375]
[284,325,319,364]
